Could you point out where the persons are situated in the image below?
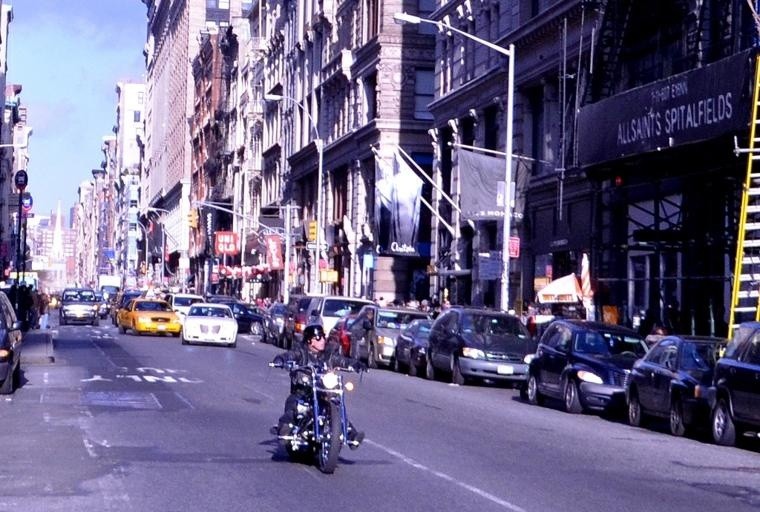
[0,279,50,351]
[508,302,587,342]
[240,295,285,316]
[360,294,457,316]
[652,326,667,336]
[271,324,369,451]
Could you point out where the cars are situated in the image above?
[623,334,730,436]
[93,285,151,328]
[180,302,241,348]
[526,319,651,414]
[203,294,268,336]
[259,295,437,378]
[0,289,24,394]
[116,298,183,338]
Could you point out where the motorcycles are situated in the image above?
[267,362,369,473]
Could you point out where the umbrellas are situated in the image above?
[581,254,594,320]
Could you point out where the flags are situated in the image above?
[457,149,529,222]
[374,156,393,256]
[135,216,343,283]
[387,151,425,256]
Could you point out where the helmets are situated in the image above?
[304,325,325,341]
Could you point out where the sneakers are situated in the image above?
[349,431,364,450]
[278,423,293,446]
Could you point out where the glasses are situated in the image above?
[312,335,325,341]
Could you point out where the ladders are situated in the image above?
[726,54,760,351]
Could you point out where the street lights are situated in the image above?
[126,217,149,276]
[264,93,323,295]
[393,12,517,314]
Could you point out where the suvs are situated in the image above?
[426,310,536,386]
[164,292,207,329]
[58,287,101,327]
[706,322,760,445]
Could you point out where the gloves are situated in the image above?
[352,361,367,373]
[273,356,284,369]
